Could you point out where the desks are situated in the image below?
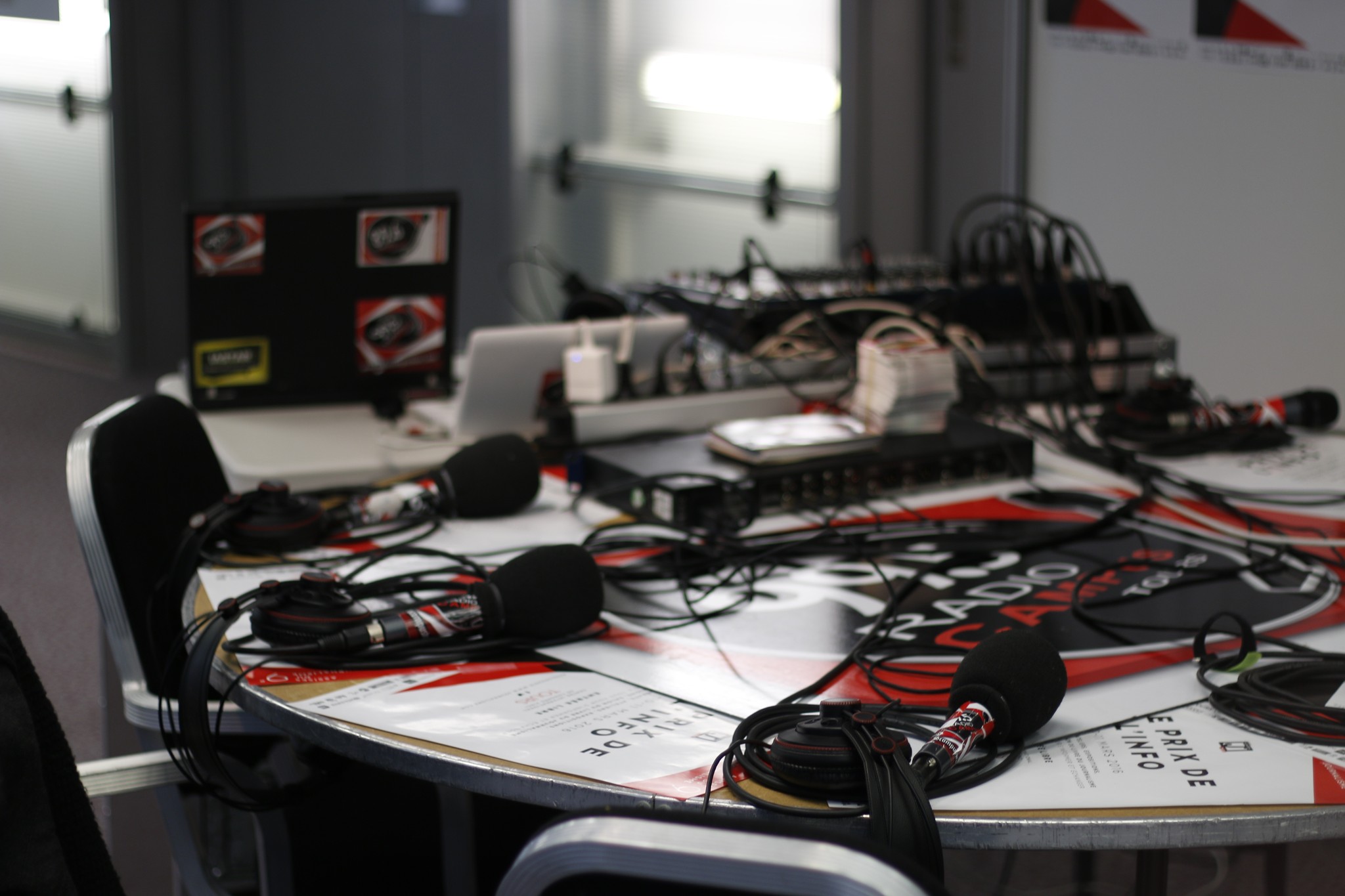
[155,352,1345,896]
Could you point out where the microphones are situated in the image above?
[327,432,541,532]
[904,630,1065,789]
[307,542,605,654]
[1214,390,1340,431]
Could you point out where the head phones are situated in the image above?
[153,567,352,813]
[155,484,327,703]
[723,703,945,895]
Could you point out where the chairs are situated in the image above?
[496,816,932,896]
[63,394,475,896]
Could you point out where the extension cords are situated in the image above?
[561,376,854,443]
